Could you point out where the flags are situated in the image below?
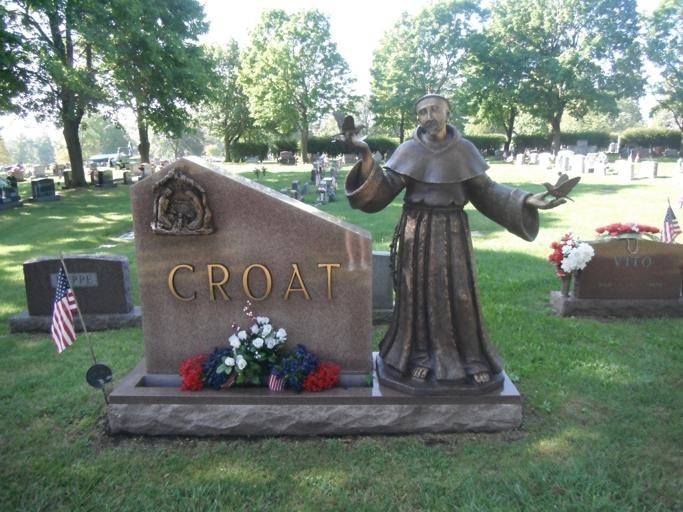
[661,205,681,243]
[51,267,78,354]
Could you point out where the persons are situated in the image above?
[185,190,204,230]
[341,93,567,383]
[156,187,172,230]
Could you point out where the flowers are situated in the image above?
[592,223,662,242]
[548,232,594,296]
[177,300,341,394]
